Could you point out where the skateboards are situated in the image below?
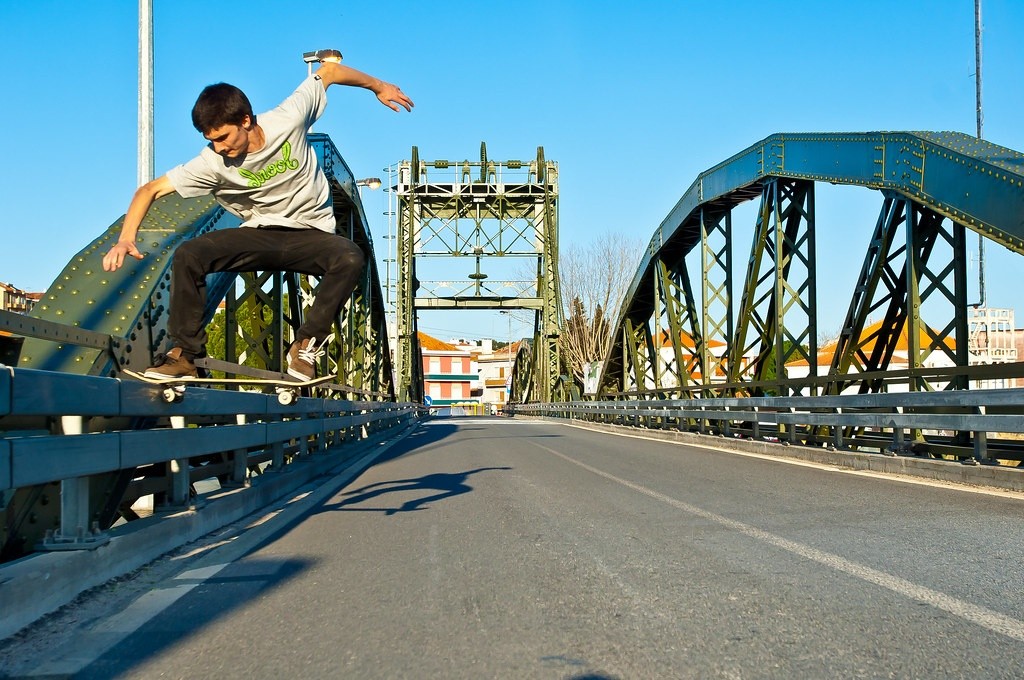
[121,367,338,405]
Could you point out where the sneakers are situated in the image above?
[286,337,325,382]
[144,347,198,381]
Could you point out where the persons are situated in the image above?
[103,62,414,382]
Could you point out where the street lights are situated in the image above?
[303,49,343,133]
[500,311,511,376]
[355,178,382,199]
